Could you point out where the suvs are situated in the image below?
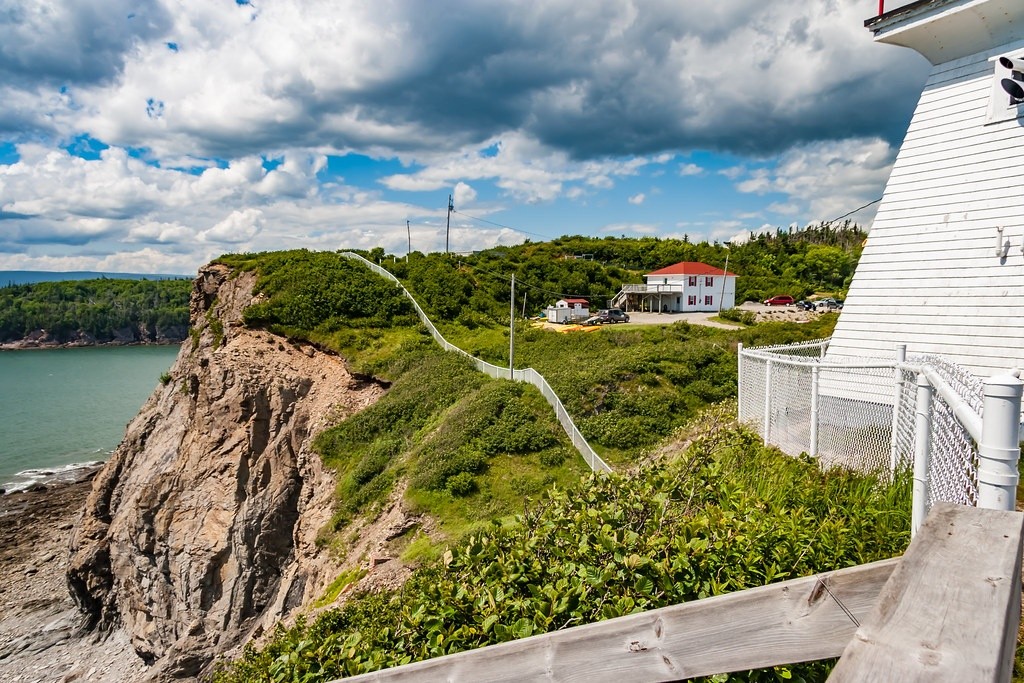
[764,295,794,307]
[598,308,630,324]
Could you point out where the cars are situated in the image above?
[813,298,845,309]
[796,300,816,311]
